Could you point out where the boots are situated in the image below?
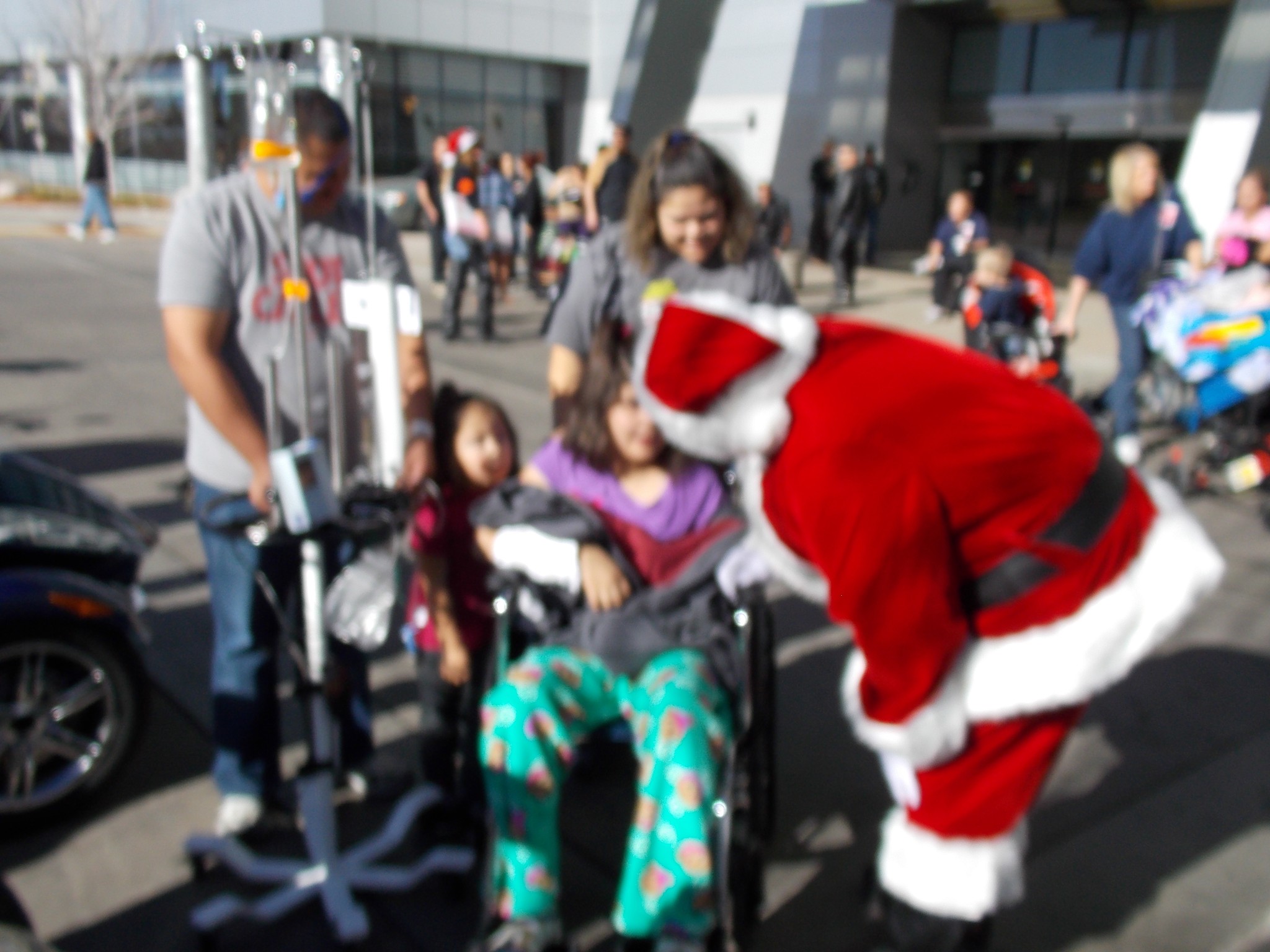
[867,887,991,952]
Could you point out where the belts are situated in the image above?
[958,441,1124,608]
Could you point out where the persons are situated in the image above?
[1054,142,1270,466]
[544,130,791,400]
[408,382,519,876]
[745,141,889,308]
[927,190,987,321]
[159,85,436,836]
[972,244,1048,365]
[69,130,116,244]
[414,122,640,342]
[637,291,1224,952]
[471,318,750,952]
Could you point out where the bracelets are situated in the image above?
[407,419,433,442]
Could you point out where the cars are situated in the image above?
[1,449,153,837]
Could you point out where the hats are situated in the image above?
[631,286,820,463]
[448,126,480,154]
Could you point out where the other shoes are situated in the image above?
[474,919,567,952]
[657,933,708,952]
[215,794,264,841]
[344,758,386,803]
[1113,433,1143,467]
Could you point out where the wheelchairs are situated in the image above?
[438,566,779,952]
